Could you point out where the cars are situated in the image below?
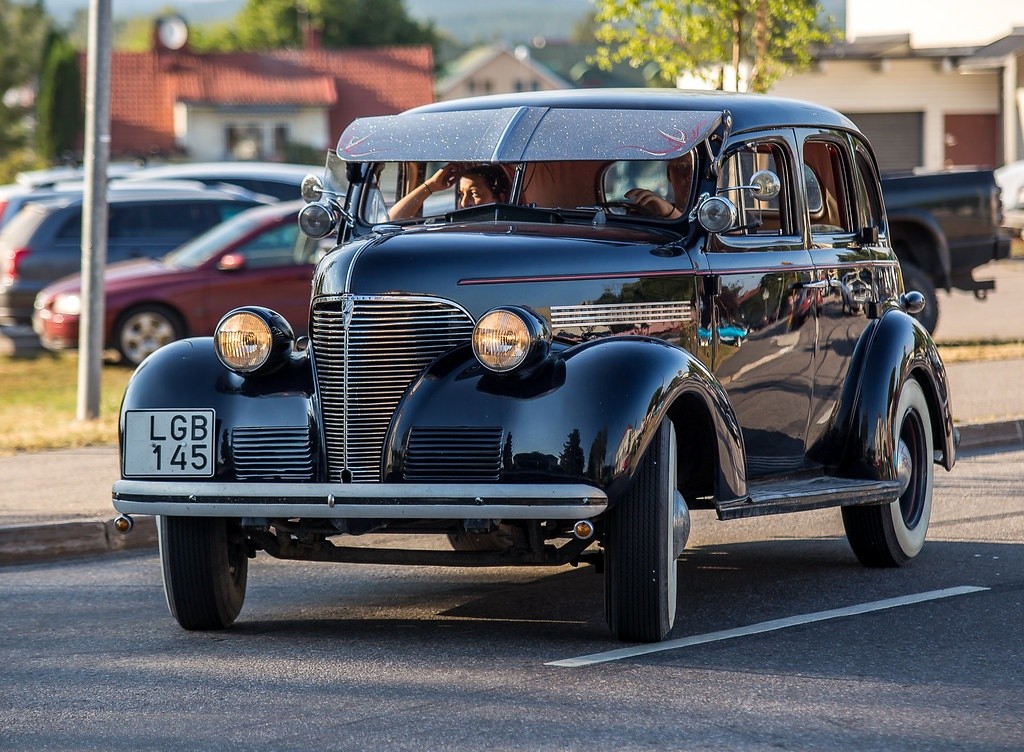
[0,161,459,368]
[993,159,1024,243]
[104,83,958,650]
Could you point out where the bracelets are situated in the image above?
[421,182,432,195]
[659,205,676,218]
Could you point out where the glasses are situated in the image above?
[666,164,692,183]
[480,162,506,192]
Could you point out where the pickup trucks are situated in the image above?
[878,148,1012,343]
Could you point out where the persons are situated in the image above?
[624,152,757,236]
[379,159,510,224]
